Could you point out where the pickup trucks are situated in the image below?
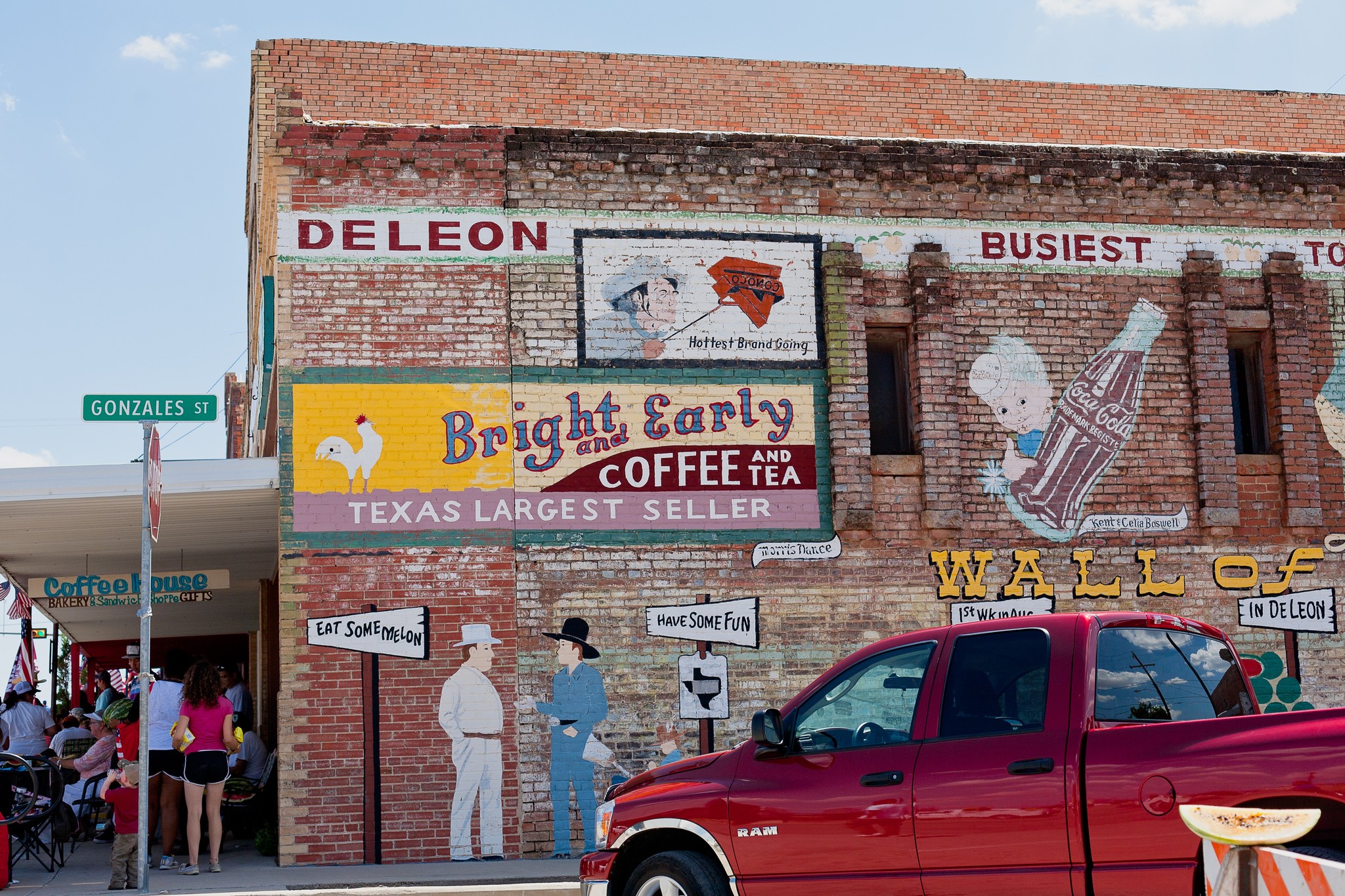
[578,610,1345,896]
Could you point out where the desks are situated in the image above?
[0,764,55,860]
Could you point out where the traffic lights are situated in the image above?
[32,628,47,639]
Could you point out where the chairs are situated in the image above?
[941,670,1041,737]
[213,750,278,855]
[8,739,115,874]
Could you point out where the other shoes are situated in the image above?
[125,884,138,889]
[93,832,115,843]
[108,884,124,890]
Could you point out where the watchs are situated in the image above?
[56,760,60,766]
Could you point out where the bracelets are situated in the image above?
[231,745,239,752]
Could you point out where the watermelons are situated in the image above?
[31,754,44,767]
[2,754,24,768]
[255,828,278,856]
[223,777,255,796]
[103,698,133,723]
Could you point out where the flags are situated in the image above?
[0,577,127,703]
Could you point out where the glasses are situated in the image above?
[216,665,226,669]
[90,722,98,724]
[69,711,73,716]
[32,694,36,697]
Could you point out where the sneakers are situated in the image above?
[148,855,154,867]
[208,864,221,873]
[177,863,199,875]
[159,854,179,870]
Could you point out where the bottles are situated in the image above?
[107,769,122,779]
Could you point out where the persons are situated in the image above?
[941,670,1003,732]
[0,646,268,890]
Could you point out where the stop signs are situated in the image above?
[147,425,164,542]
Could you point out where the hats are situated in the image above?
[118,758,139,786]
[68,707,85,721]
[83,712,102,721]
[13,681,41,695]
[95,671,111,680]
[122,641,140,659]
